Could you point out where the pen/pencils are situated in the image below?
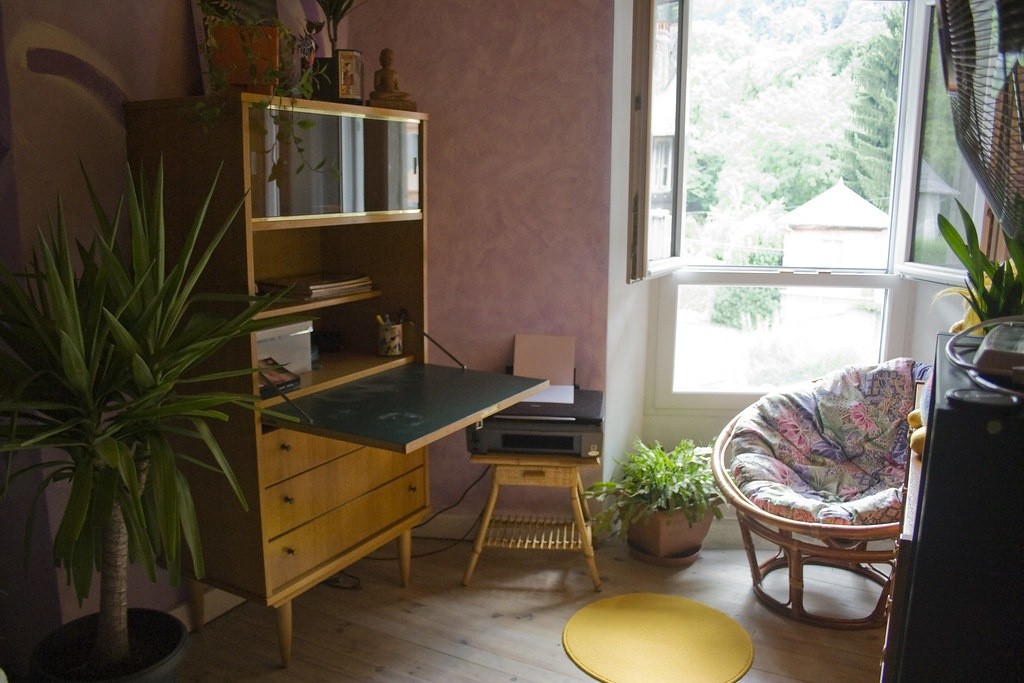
[376,314,405,324]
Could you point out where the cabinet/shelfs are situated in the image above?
[119,91,549,669]
[896,334,1024,683]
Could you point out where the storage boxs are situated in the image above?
[252,317,313,383]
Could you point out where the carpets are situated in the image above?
[563,592,755,683]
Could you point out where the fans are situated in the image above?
[930,0,1024,382]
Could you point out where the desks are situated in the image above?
[460,454,602,594]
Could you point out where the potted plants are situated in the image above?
[178,0,342,185]
[0,151,319,683]
[582,436,732,566]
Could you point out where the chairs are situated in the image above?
[713,358,933,631]
